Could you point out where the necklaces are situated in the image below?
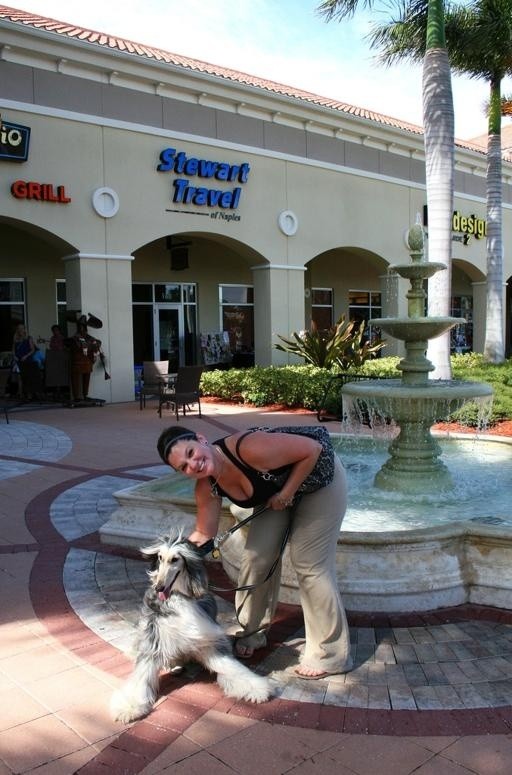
[209,447,226,498]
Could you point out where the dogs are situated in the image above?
[110,522,278,727]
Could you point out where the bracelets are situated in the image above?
[275,494,295,510]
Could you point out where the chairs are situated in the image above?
[0,349,74,425]
[136,361,204,421]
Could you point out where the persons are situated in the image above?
[32,344,46,381]
[153,423,355,680]
[12,323,37,400]
[57,315,102,402]
[49,325,66,349]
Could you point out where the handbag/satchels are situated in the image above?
[236,423,335,495]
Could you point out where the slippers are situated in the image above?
[293,664,353,680]
[232,638,254,658]
